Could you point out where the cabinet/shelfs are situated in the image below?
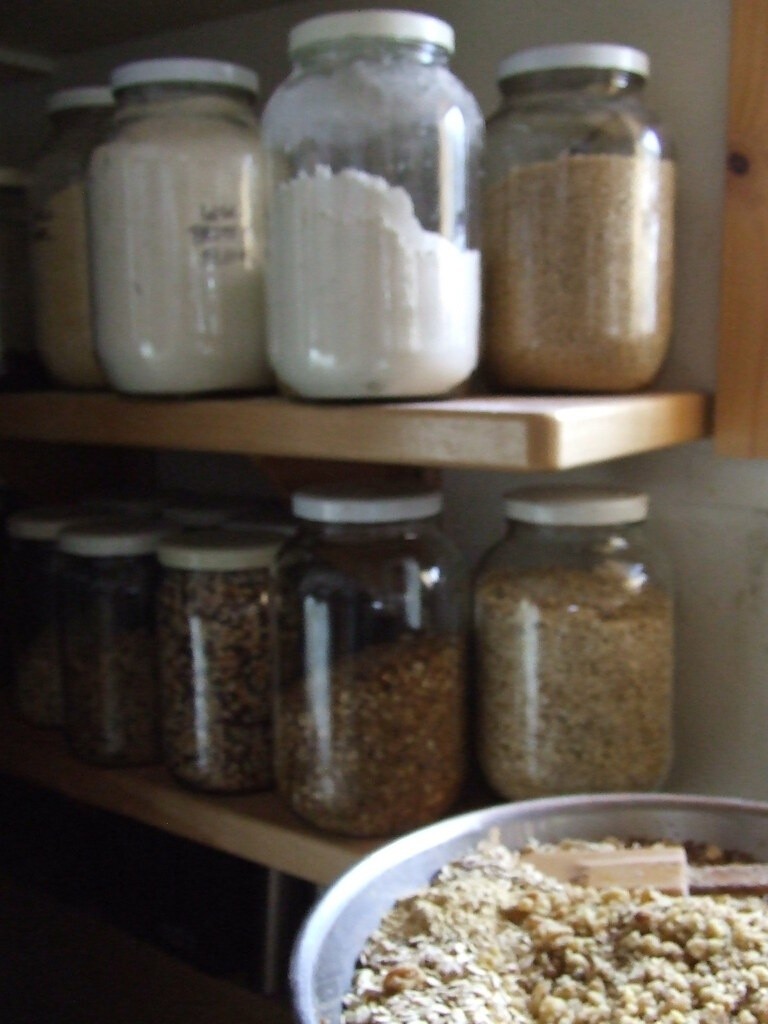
[0,380,716,1024]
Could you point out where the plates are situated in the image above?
[296,795,768,1024]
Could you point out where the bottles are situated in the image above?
[85,56,264,396]
[472,488,678,800]
[483,43,675,388]
[31,88,114,392]
[0,494,270,790]
[259,11,486,400]
[272,483,468,839]
